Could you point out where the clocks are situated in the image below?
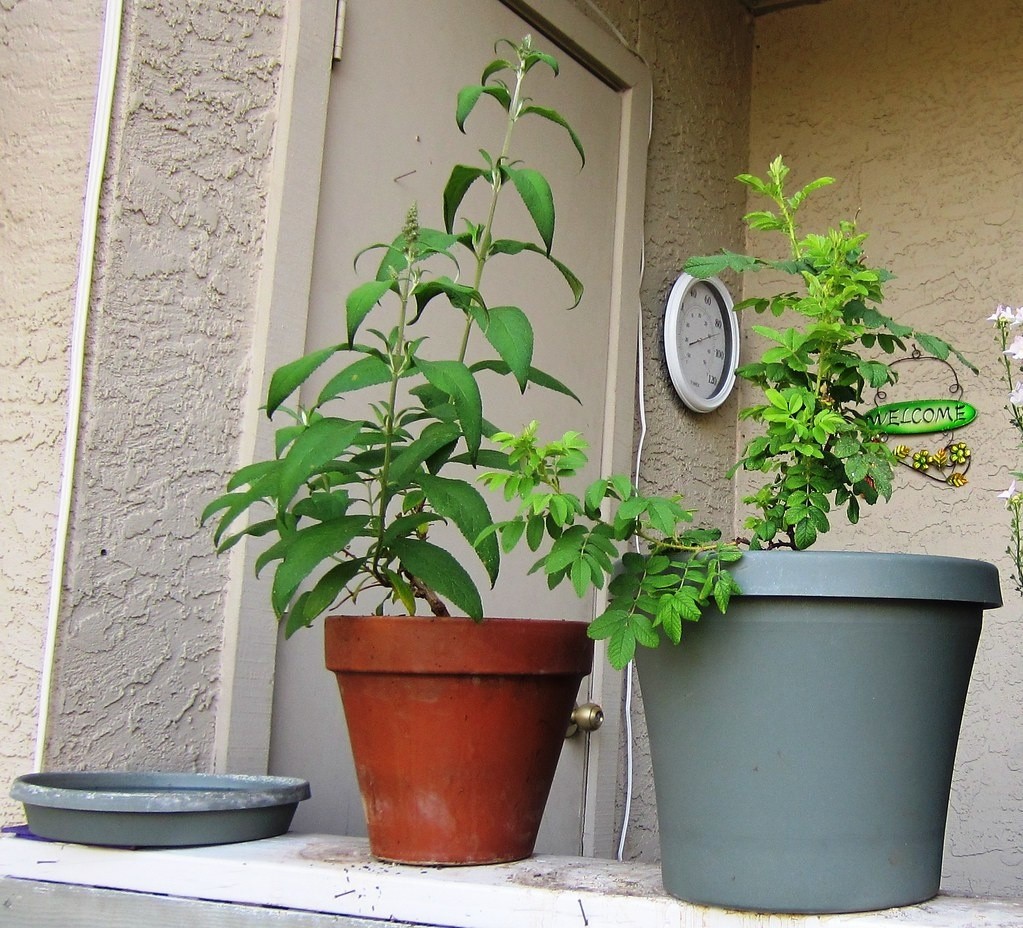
[662,269,740,416]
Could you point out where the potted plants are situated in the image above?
[194,30,594,872]
[473,150,1008,912]
